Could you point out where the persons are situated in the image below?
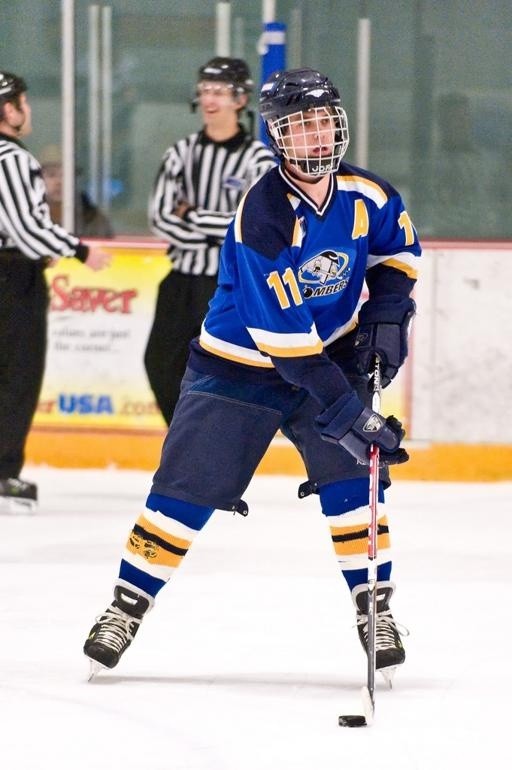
[84,70,421,672]
[141,55,281,434]
[36,144,115,241]
[0,67,117,503]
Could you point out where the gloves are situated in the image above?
[311,388,410,469]
[352,294,417,393]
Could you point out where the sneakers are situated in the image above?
[1,477,39,501]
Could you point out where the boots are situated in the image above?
[350,580,407,671]
[82,576,157,671]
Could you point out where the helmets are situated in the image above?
[257,66,351,179]
[0,70,29,121]
[189,56,255,115]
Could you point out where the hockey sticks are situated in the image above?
[339,351,383,727]
